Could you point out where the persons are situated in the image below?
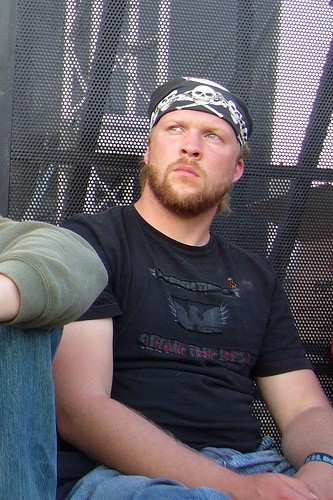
[0,211,111,500]
[46,74,333,500]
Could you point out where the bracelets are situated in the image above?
[303,451,333,465]
